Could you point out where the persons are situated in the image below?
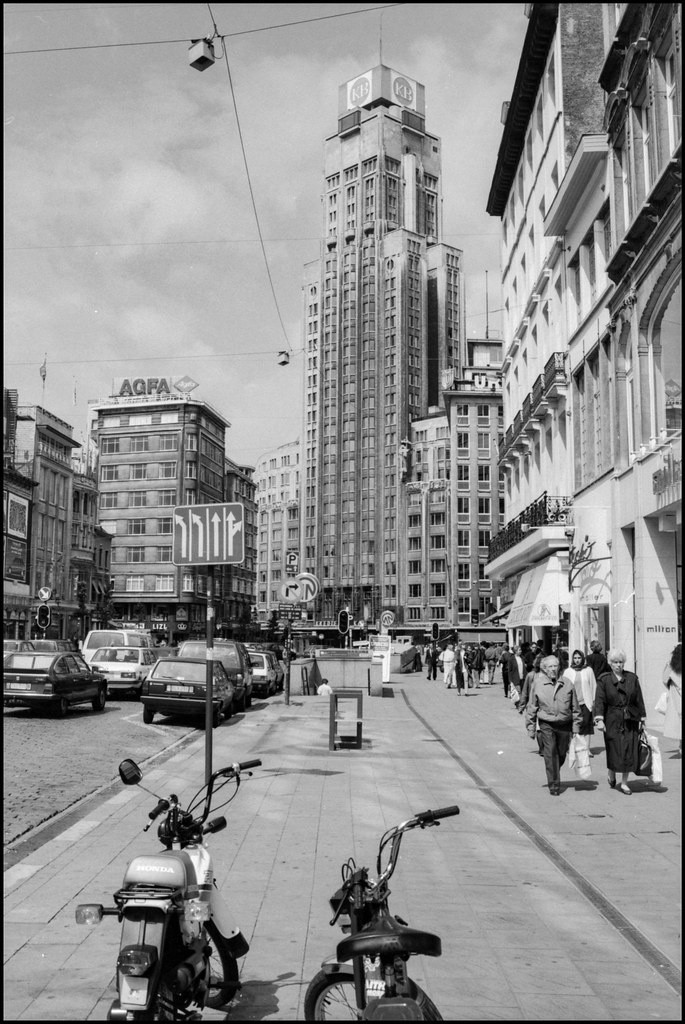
[594,648,647,794]
[586,640,608,678]
[518,650,547,756]
[526,655,584,796]
[553,643,569,672]
[662,643,682,753]
[109,650,118,662]
[425,639,544,709]
[563,649,597,758]
[317,679,332,695]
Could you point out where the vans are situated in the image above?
[83,629,153,668]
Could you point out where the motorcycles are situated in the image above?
[75,758,263,1023]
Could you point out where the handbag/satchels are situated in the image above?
[568,733,591,781]
[635,721,663,784]
[510,683,520,704]
[655,692,668,715]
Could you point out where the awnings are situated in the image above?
[507,556,560,628]
[482,602,512,623]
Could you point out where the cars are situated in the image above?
[140,657,238,729]
[153,646,181,662]
[88,647,158,700]
[2,639,78,667]
[240,638,297,692]
[2,650,109,717]
[303,643,330,658]
[248,651,278,700]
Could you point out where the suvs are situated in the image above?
[178,642,261,712]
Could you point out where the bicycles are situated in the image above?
[304,803,462,1022]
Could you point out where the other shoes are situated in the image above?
[607,772,616,787]
[550,789,559,795]
[620,786,632,795]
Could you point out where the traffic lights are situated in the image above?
[338,610,349,635]
[432,622,441,640]
[37,605,51,629]
